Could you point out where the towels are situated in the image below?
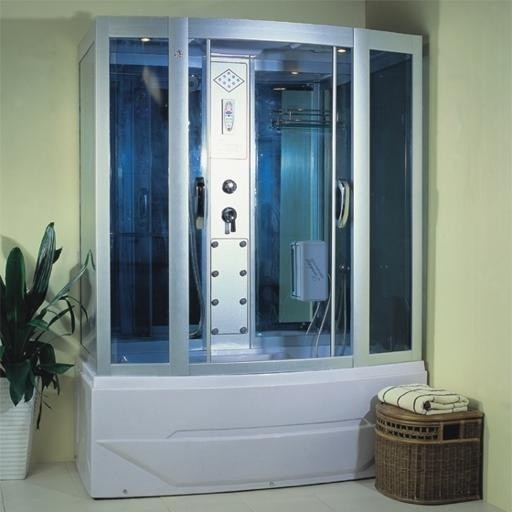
[376,381,469,414]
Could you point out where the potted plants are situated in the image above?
[0,220,94,479]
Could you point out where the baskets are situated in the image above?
[375,401,485,507]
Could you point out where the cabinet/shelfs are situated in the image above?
[70,15,428,497]
